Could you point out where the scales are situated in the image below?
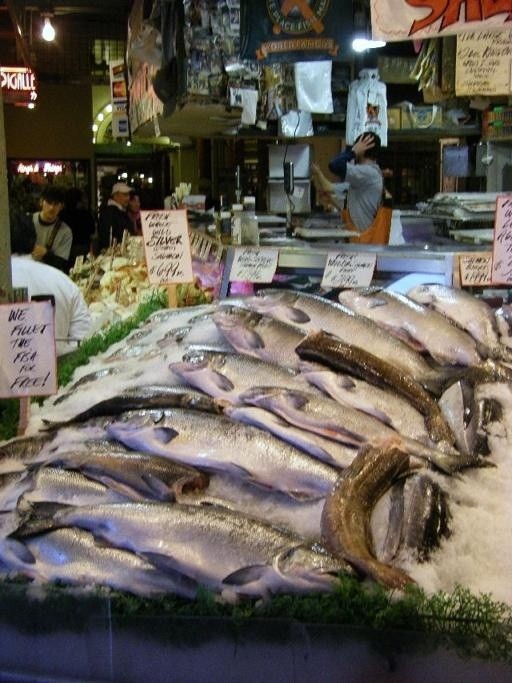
[283,161,361,243]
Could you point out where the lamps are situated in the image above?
[40,8,57,42]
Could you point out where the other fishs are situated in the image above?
[0,282,512,614]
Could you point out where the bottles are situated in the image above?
[219,211,230,243]
[241,193,259,244]
[230,202,244,243]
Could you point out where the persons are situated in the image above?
[57,186,95,254]
[15,187,73,272]
[329,131,392,244]
[97,182,133,252]
[128,194,145,238]
[8,208,91,354]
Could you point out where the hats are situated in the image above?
[111,181,132,194]
